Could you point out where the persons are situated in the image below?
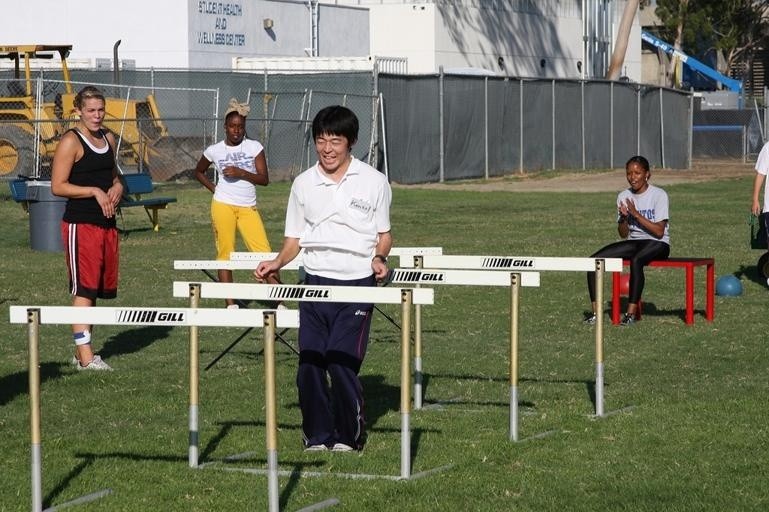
[584,154,671,326]
[253,105,392,451]
[196,99,286,310]
[52,85,124,371]
[751,140,769,284]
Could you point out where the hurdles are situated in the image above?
[398,252,638,419]
[178,257,446,414]
[10,305,340,511]
[229,247,472,404]
[171,279,459,483]
[379,266,563,444]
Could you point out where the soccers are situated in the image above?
[716,273,743,298]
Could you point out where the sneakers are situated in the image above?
[620,313,636,326]
[73,355,112,370]
[332,444,353,452]
[304,443,327,453]
[582,313,596,324]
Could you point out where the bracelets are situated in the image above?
[373,252,387,264]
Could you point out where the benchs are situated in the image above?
[603,252,718,328]
[10,172,180,236]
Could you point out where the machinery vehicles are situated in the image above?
[0,38,216,185]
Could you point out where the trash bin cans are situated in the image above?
[25,181,69,250]
